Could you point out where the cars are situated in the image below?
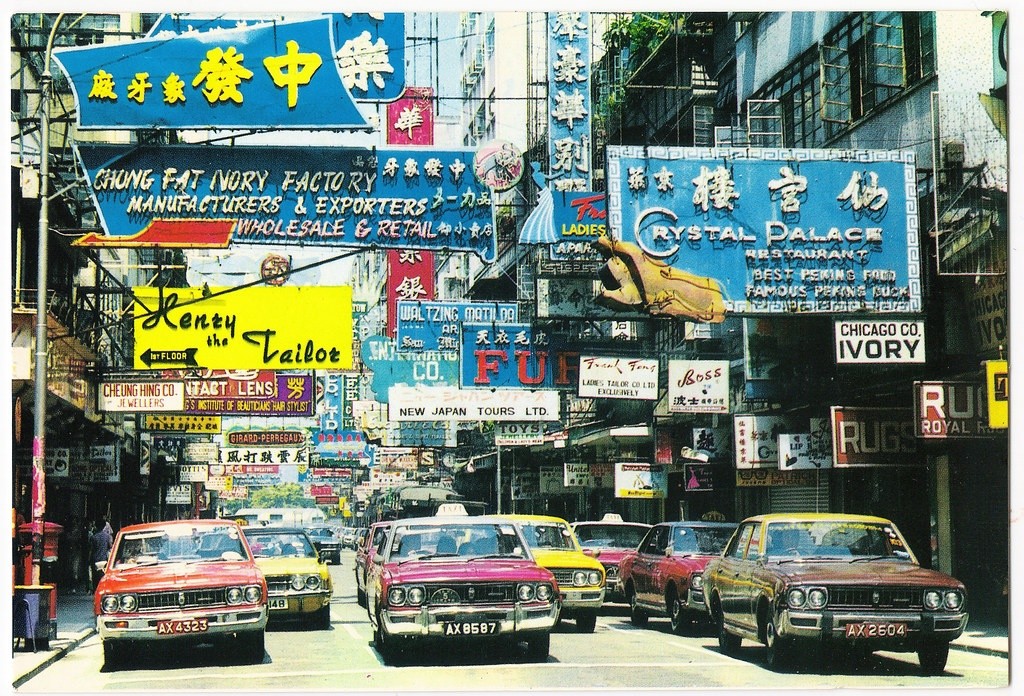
[465,514,607,635]
[92,518,271,665]
[338,519,474,610]
[230,524,333,629]
[367,502,563,664]
[299,522,343,566]
[565,512,653,603]
[619,511,751,636]
[701,512,970,675]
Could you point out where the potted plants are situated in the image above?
[495,206,515,235]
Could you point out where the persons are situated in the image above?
[82,512,115,597]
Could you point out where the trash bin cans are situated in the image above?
[14,586,55,653]
